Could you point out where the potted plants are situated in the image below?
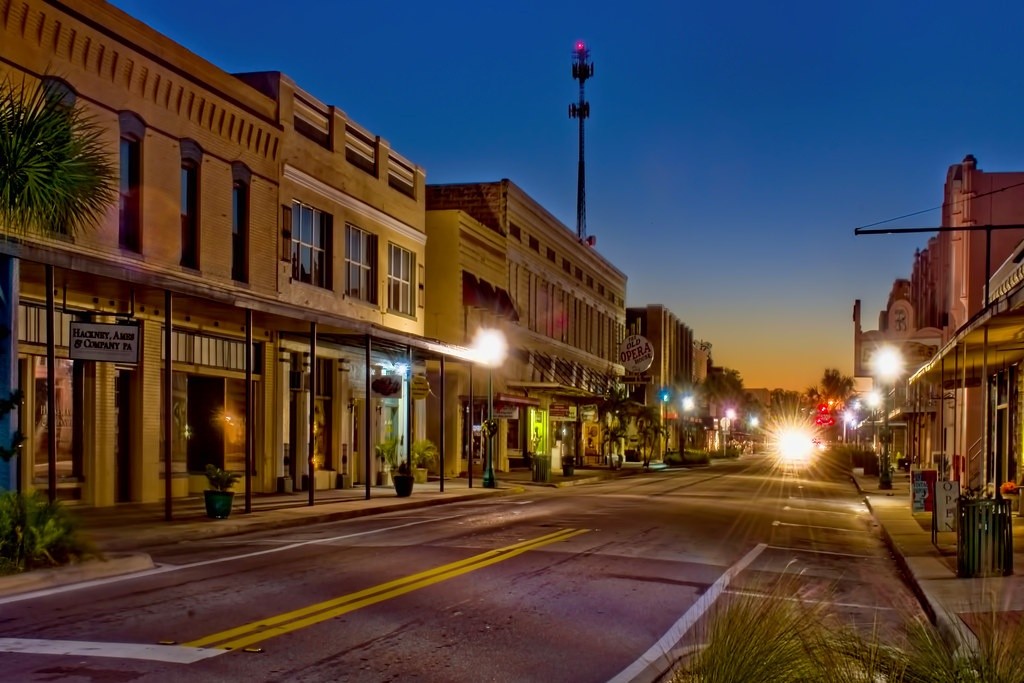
[414,438,439,482]
[392,461,414,497]
[201,465,239,518]
[562,455,575,476]
[896,452,907,469]
[375,443,398,486]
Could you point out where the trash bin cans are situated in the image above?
[529,455,547,481]
[954,498,1012,577]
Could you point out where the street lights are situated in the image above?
[680,397,692,454]
[878,347,897,489]
[478,332,498,488]
